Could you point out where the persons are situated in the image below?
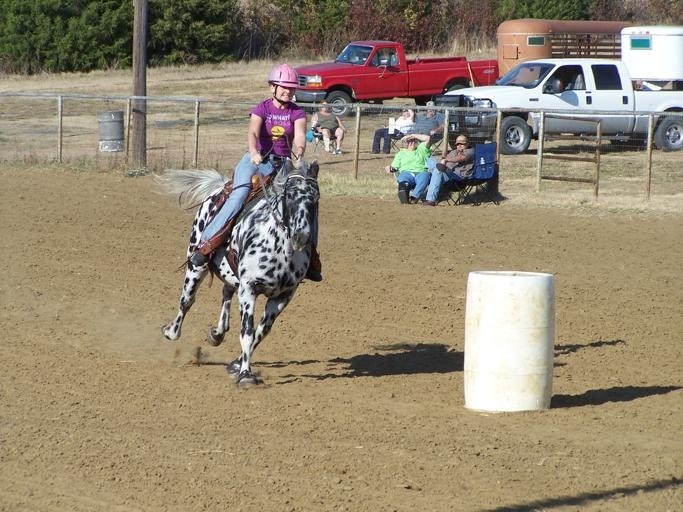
[369,104,415,154]
[190,63,308,267]
[311,99,347,154]
[422,135,475,206]
[384,134,432,205]
[411,100,444,136]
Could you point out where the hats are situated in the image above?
[454,135,468,145]
[426,101,434,106]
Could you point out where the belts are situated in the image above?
[263,155,285,161]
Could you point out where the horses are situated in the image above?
[150,156,321,388]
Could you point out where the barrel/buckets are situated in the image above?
[463,270,555,413]
[97,110,125,154]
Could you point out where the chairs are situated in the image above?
[366,52,380,68]
[313,131,344,153]
[385,52,396,67]
[564,73,584,91]
[456,142,500,206]
[406,112,444,158]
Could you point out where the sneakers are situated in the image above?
[191,243,209,266]
[398,190,408,203]
[422,201,435,206]
[329,149,336,154]
[336,149,343,154]
[306,266,322,282]
[436,163,453,175]
[409,196,417,203]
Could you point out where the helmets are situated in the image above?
[268,63,302,88]
